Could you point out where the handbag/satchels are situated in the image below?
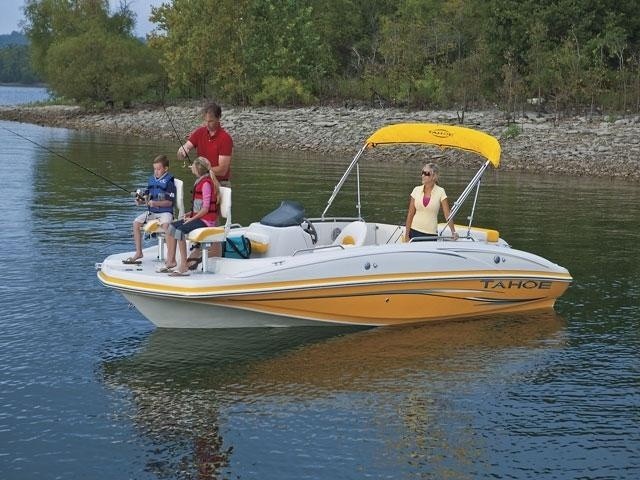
[223,235,251,259]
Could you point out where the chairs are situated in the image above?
[149,178,233,276]
[313,219,367,253]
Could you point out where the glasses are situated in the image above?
[420,170,431,176]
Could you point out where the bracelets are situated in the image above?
[452,232,457,234]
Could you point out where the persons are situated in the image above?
[156,157,220,277]
[177,102,233,187]
[404,163,459,242]
[131,154,177,265]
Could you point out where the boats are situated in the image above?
[94,122,574,330]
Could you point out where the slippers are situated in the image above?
[169,270,190,276]
[156,264,173,273]
[122,257,142,264]
[186,257,202,270]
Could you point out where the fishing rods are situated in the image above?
[0,127,145,201]
[155,91,193,165]
